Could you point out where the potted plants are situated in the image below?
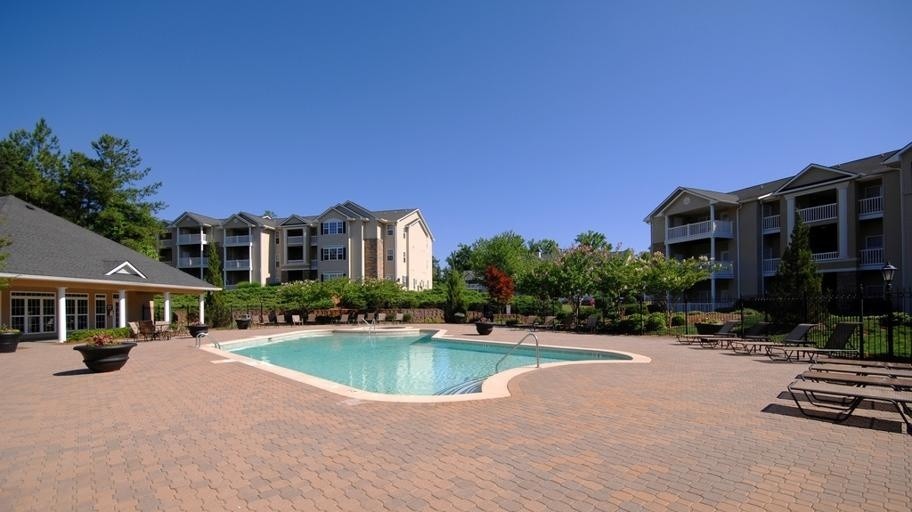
[475,316,497,335]
[0,323,26,353]
[184,319,210,337]
[73,331,137,372]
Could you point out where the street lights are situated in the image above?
[881,261,899,361]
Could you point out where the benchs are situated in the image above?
[785,355,912,435]
[513,323,554,332]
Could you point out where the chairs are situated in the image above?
[127,319,183,342]
[575,316,601,334]
[335,312,404,325]
[251,313,316,328]
[676,318,864,363]
